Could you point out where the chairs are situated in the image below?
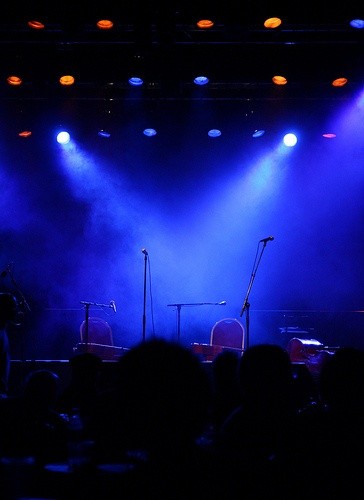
[80,317,113,345]
[210,318,245,354]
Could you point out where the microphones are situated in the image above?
[260,237,274,242]
[142,248,148,255]
[216,300,227,305]
[109,300,117,312]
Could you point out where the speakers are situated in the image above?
[190,343,243,362]
[76,343,128,361]
[287,338,323,362]
